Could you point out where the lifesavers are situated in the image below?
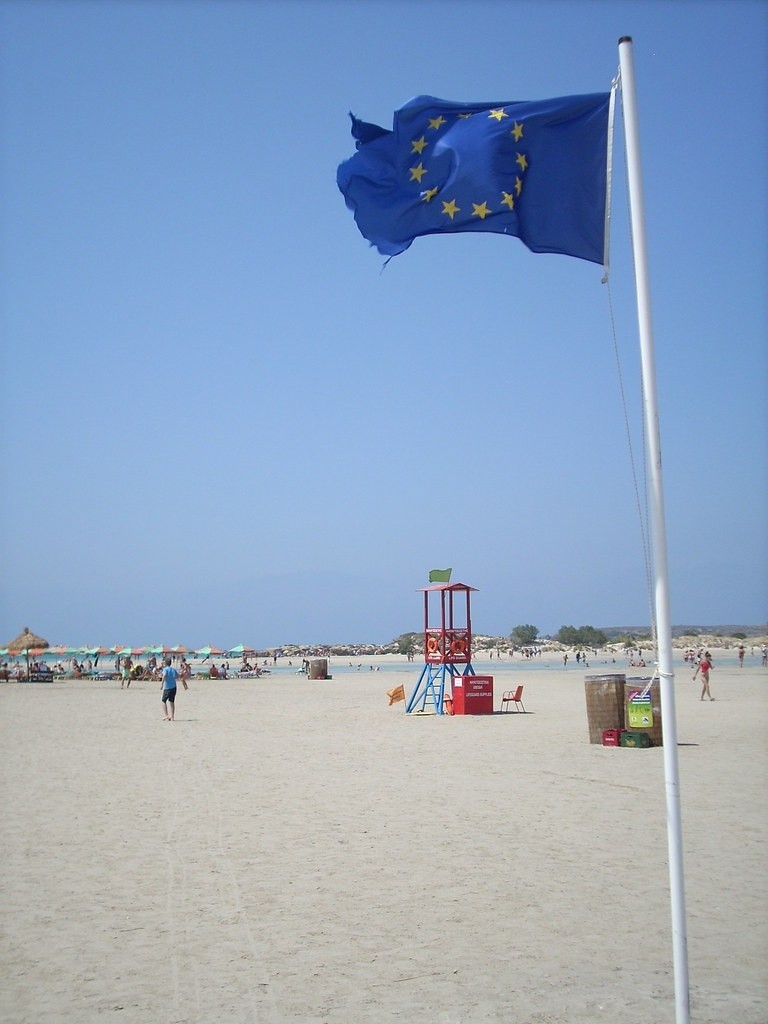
[452,640,465,653]
[427,638,438,652]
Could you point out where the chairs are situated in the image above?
[8,671,231,683]
[234,667,261,678]
[501,686,526,713]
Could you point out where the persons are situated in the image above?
[563,648,703,668]
[473,646,542,660]
[210,661,229,680]
[241,652,414,673]
[739,644,768,668]
[159,658,181,722]
[85,656,191,691]
[692,651,716,703]
[0,653,85,678]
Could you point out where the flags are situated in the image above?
[428,568,452,583]
[333,90,615,276]
[386,685,406,707]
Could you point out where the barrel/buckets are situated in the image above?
[311,659,327,679]
[585,674,626,744]
[623,677,662,747]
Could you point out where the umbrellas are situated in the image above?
[229,643,255,665]
[194,645,224,666]
[1,644,190,655]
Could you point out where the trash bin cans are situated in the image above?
[582,672,626,745]
[622,676,663,747]
[310,658,328,679]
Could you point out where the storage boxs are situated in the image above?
[602,728,650,748]
